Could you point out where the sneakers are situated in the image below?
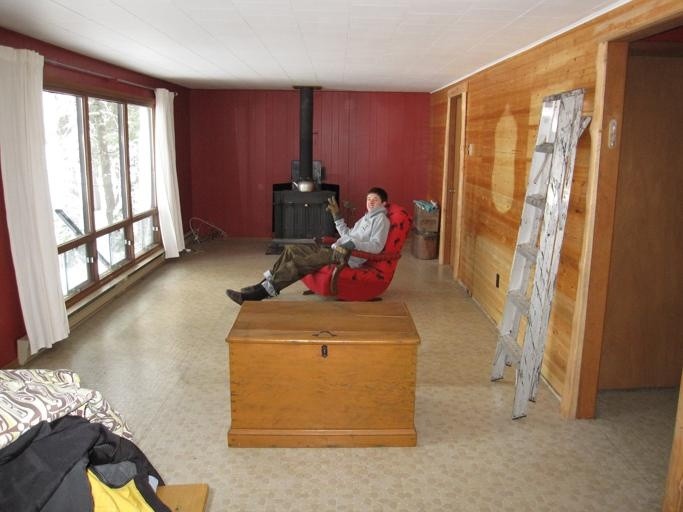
[240,285,254,293]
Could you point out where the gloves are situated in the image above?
[325,196,342,222]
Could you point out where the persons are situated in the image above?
[226,187,391,306]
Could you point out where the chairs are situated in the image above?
[302,202,411,301]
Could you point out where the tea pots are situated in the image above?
[292,176,315,193]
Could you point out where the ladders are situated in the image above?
[505,87,591,403]
[491,88,573,423]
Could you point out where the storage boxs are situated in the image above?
[412,230,438,260]
[415,204,439,233]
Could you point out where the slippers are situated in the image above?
[331,241,355,265]
[226,289,243,305]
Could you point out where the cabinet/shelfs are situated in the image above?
[225,300,421,448]
